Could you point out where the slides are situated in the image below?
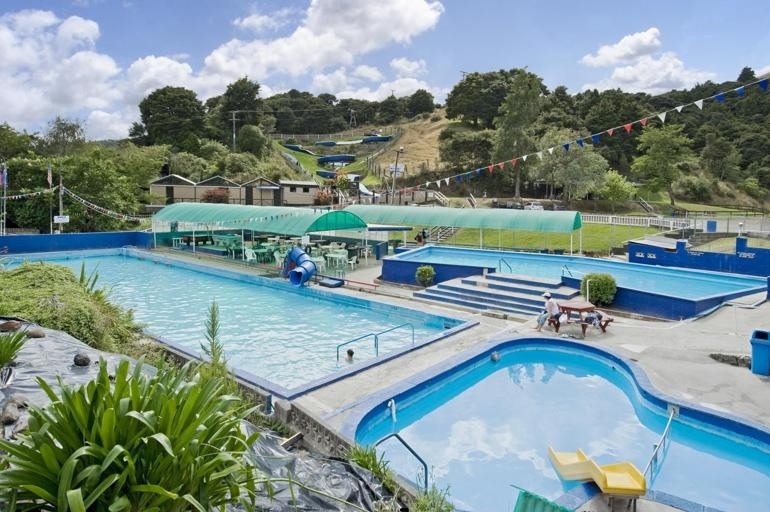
[550,447,645,495]
[284,247,316,287]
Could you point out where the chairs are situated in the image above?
[245,236,358,279]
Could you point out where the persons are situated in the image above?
[346,349,354,360]
[416,228,428,247]
[533,293,558,332]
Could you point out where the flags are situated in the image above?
[47,164,53,185]
[0,168,8,187]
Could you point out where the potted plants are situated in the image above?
[0,322,33,389]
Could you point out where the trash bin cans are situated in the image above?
[748,329,770,376]
